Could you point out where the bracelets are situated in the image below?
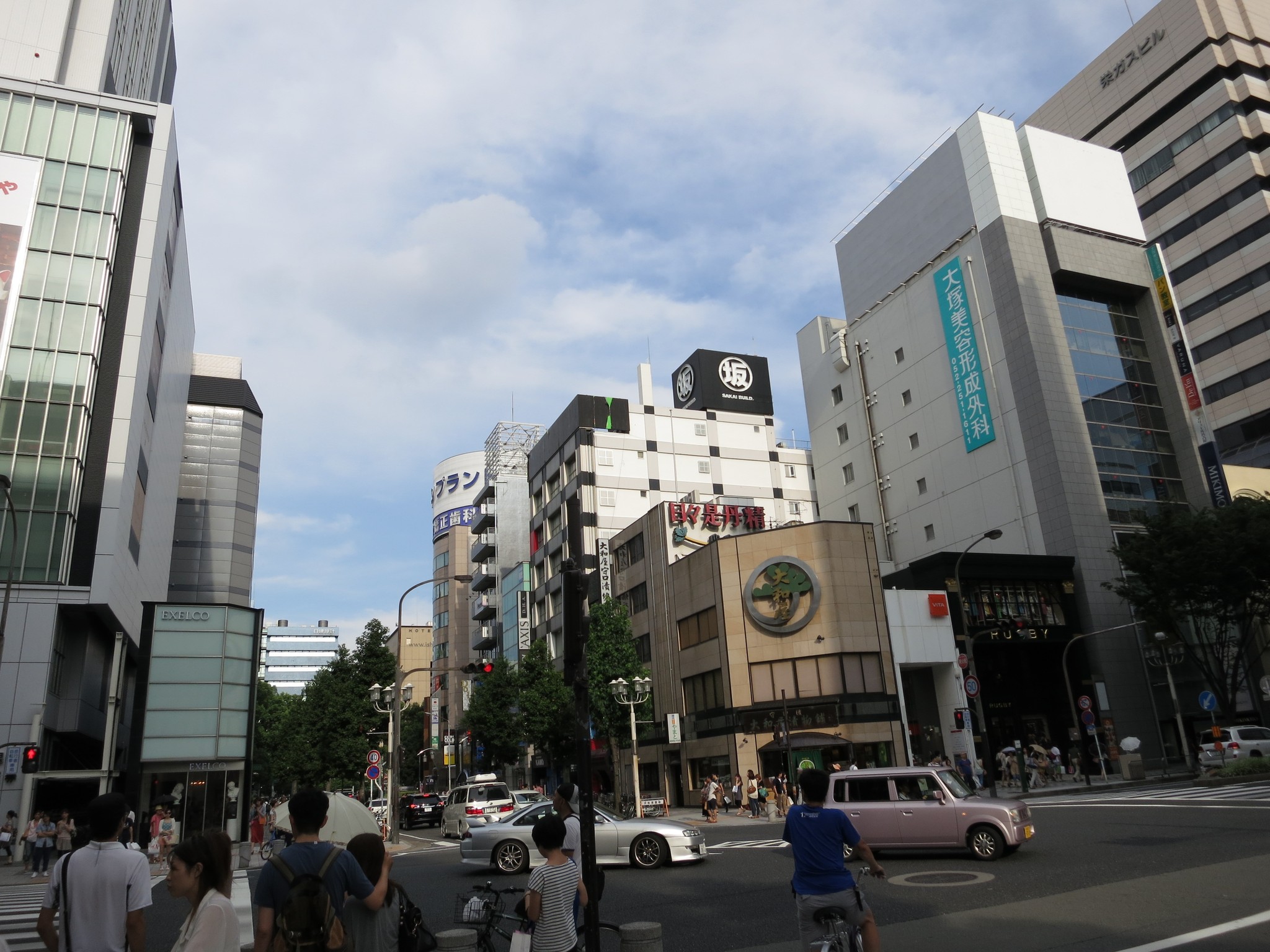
[785,790,787,792]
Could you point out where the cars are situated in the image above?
[459,799,709,870]
[510,790,558,819]
[1197,724,1270,765]
[365,792,449,829]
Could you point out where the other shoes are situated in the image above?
[980,775,1084,791]
[705,806,788,823]
[251,851,254,855]
[149,857,170,870]
[4,860,49,878]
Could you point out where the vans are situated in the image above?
[441,773,514,840]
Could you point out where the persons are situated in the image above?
[524,815,588,952]
[1127,750,1136,754]
[521,783,543,794]
[37,792,240,952]
[733,769,767,818]
[833,758,858,773]
[773,770,788,818]
[897,780,913,799]
[929,747,1110,791]
[552,782,582,928]
[701,770,725,823]
[250,795,293,855]
[253,786,410,952]
[0,810,75,877]
[796,767,803,774]
[782,768,886,952]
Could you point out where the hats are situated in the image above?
[557,782,579,813]
[155,805,162,811]
[960,752,965,755]
[833,763,841,772]
[796,767,803,771]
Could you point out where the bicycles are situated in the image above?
[260,823,292,860]
[593,791,661,818]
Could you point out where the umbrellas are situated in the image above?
[270,791,385,849]
[1120,736,1141,750]
[1002,744,1048,755]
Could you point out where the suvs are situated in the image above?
[825,769,1034,861]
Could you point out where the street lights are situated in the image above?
[368,681,415,839]
[1061,620,1148,785]
[392,574,474,837]
[1141,632,1192,767]
[609,677,652,819]
[953,528,1004,797]
[425,712,452,793]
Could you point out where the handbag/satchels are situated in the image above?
[148,838,160,855]
[23,828,29,838]
[1057,766,1066,774]
[163,835,177,845]
[390,880,438,952]
[514,894,530,919]
[0,832,11,842]
[71,827,77,838]
[126,842,141,852]
[1037,761,1048,768]
[510,909,531,952]
[259,814,266,825]
[1069,765,1075,773]
[700,779,794,810]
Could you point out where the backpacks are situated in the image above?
[269,846,355,952]
[996,753,1003,767]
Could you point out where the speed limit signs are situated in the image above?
[366,749,382,764]
[964,674,979,698]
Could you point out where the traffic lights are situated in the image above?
[954,711,966,731]
[21,746,39,774]
[462,662,495,675]
[995,618,1026,630]
[772,725,781,745]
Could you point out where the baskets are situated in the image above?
[453,893,506,924]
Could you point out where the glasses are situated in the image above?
[166,812,171,815]
